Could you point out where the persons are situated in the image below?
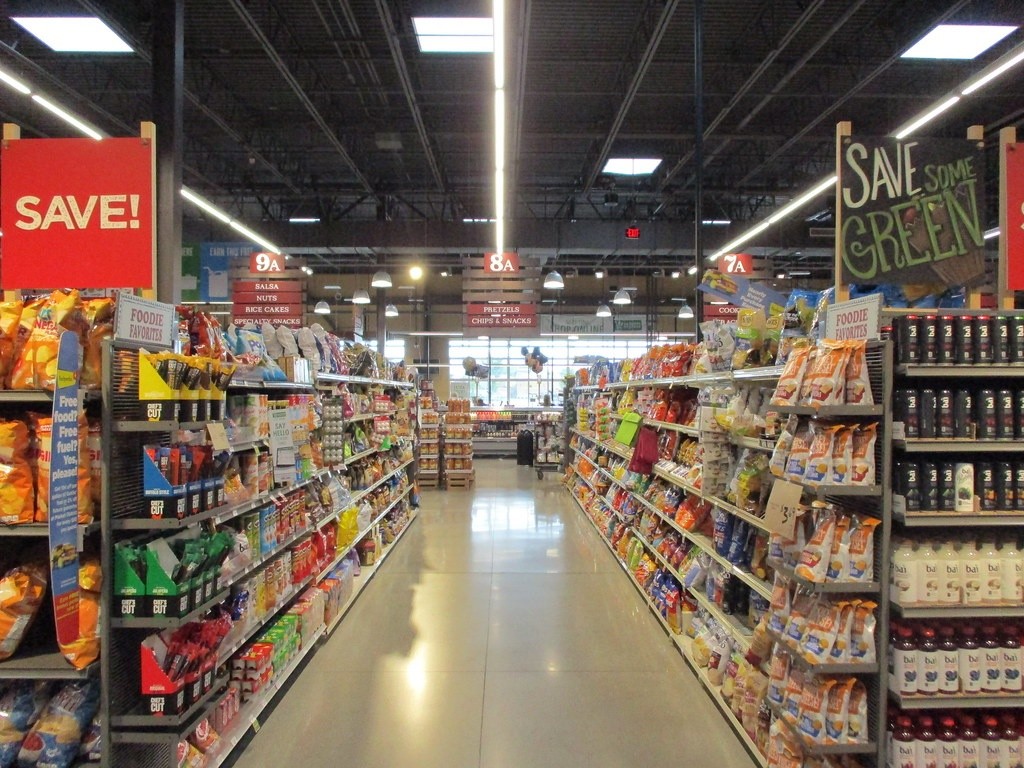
[477,399,487,406]
[540,394,556,406]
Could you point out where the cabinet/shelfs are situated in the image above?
[0,343,476,768]
[532,303,1024,768]
[471,414,529,458]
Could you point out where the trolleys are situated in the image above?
[534,418,563,480]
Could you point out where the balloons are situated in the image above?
[521,345,548,374]
[463,356,476,371]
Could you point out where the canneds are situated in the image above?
[880,314,1024,513]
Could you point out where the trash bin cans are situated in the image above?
[516,429,534,465]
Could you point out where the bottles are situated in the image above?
[881,534,1024,767]
[882,310,1024,515]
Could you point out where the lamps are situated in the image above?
[985,227,1001,240]
[0,64,400,319]
[542,190,812,319]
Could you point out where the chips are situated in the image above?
[0,482,26,515]
[26,346,57,380]
